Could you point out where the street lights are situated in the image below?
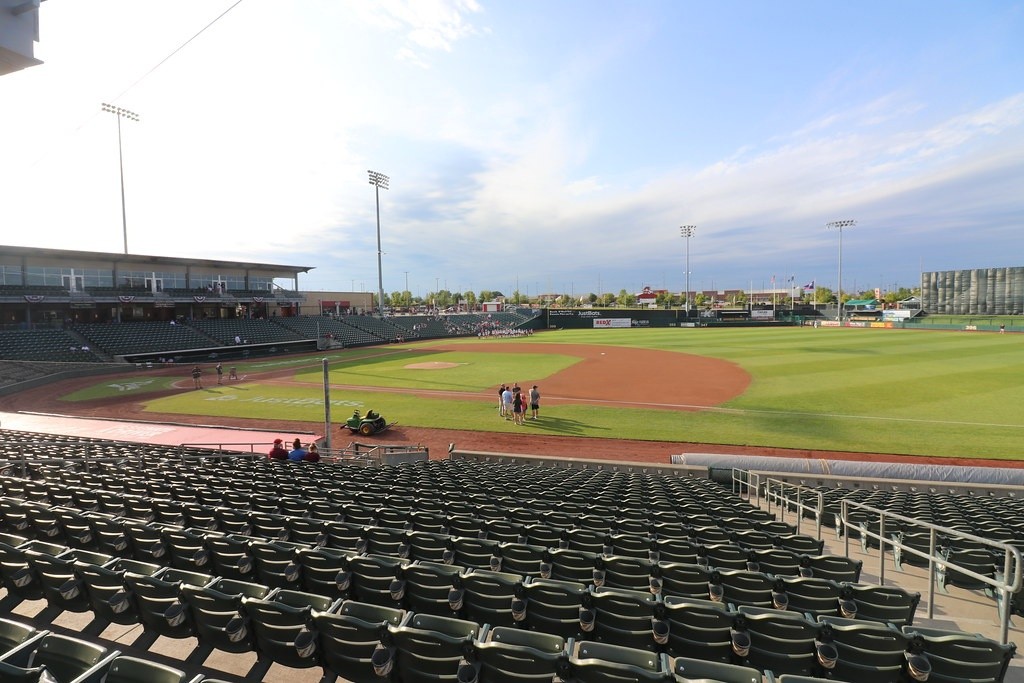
[825,220,854,322]
[101,103,139,253]
[679,225,697,316]
[367,170,390,306]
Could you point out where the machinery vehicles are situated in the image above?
[340,409,399,437]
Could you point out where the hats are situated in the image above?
[274,439,282,444]
[533,385,538,388]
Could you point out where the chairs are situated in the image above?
[0,285,1024,683]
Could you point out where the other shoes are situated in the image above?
[499,412,535,425]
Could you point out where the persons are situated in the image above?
[1000,323,1005,333]
[814,321,817,328]
[529,385,540,418]
[272,310,275,317]
[304,442,320,463]
[216,362,223,383]
[288,439,305,460]
[206,282,225,293]
[170,314,185,325]
[269,439,289,459]
[81,344,89,350]
[235,334,240,344]
[203,312,207,319]
[498,383,527,425]
[192,366,203,389]
[322,307,533,344]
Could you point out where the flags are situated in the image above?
[788,275,794,282]
[804,280,814,290]
[771,275,775,283]
